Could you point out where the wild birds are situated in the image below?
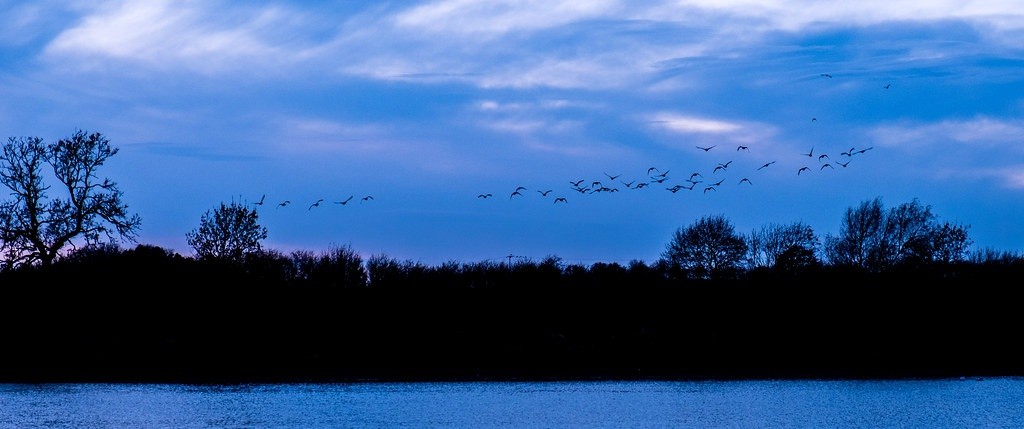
[477,145,875,204]
[812,117,817,122]
[821,73,833,78]
[884,83,890,89]
[251,194,374,212]
